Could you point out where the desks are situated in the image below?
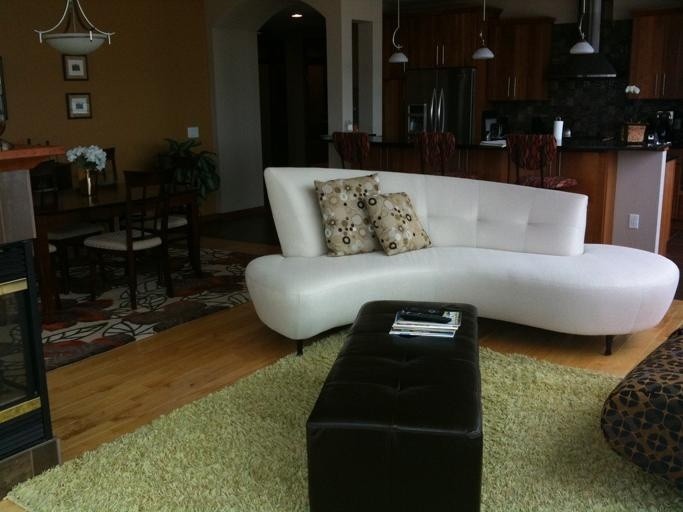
[30,183,197,325]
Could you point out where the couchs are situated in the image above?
[243,165,680,357]
[601,323,683,489]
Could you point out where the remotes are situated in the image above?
[401,311,451,325]
[402,304,445,316]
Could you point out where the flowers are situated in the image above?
[66,143,108,173]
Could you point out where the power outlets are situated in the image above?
[628,213,640,228]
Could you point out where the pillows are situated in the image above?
[362,191,432,256]
[313,172,381,257]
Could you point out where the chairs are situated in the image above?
[505,133,578,190]
[416,131,456,176]
[28,162,104,307]
[84,170,177,309]
[331,130,371,170]
[119,155,201,277]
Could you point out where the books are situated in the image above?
[389,310,462,337]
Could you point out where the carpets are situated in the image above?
[4,328,683,512]
[0,242,264,384]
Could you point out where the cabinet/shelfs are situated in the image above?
[0,141,66,501]
[486,16,557,102]
[626,7,683,101]
[405,5,502,71]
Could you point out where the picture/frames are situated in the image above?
[65,92,92,119]
[62,54,88,81]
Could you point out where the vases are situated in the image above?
[80,168,97,196]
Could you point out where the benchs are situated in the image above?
[305,300,483,512]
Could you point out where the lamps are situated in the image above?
[33,0,116,57]
[387,0,409,64]
[472,1,495,60]
[569,0,595,55]
